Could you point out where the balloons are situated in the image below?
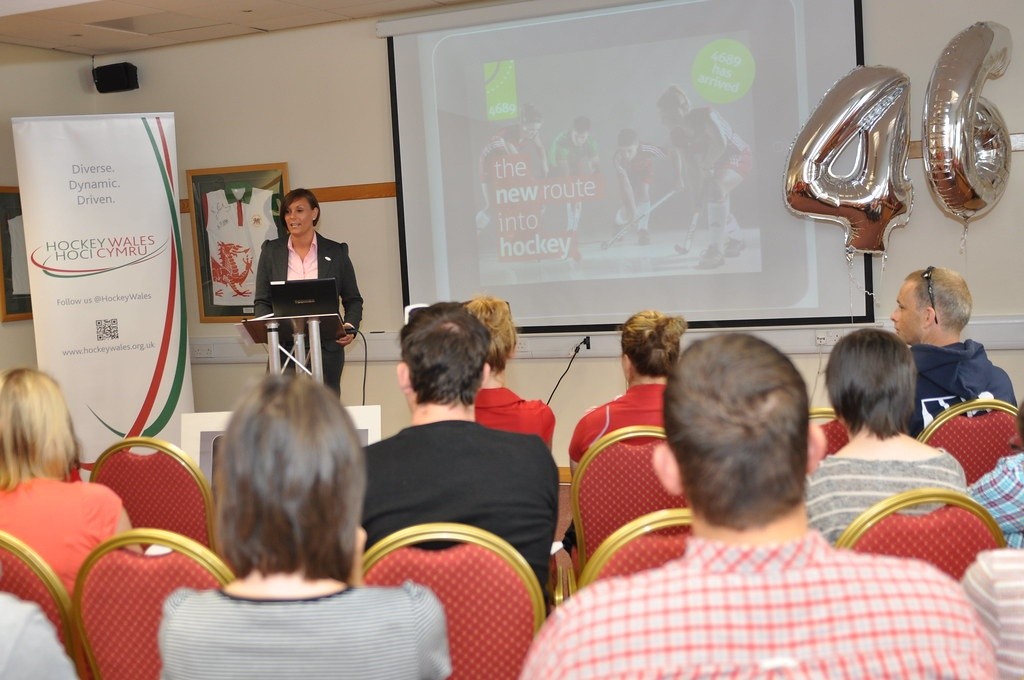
[785,66,915,254]
[922,22,1012,227]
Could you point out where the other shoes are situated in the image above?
[694,244,723,269]
[637,229,649,244]
[721,239,747,257]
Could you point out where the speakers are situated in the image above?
[93,62,139,94]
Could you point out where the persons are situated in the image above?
[254,188,363,400]
[359,304,560,618]
[805,328,965,548]
[890,268,1016,440]
[519,333,995,679]
[562,311,687,554]
[0,595,78,680]
[477,88,755,268]
[962,403,1024,680]
[1,366,143,599]
[158,377,453,680]
[463,297,556,452]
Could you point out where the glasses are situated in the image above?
[922,265,940,325]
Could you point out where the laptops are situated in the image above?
[270,277,345,326]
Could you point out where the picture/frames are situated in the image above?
[186,161,292,322]
[0,187,36,320]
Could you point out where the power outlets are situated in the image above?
[815,329,842,346]
[516,338,530,351]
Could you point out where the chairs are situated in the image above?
[1,403,1024,680]
